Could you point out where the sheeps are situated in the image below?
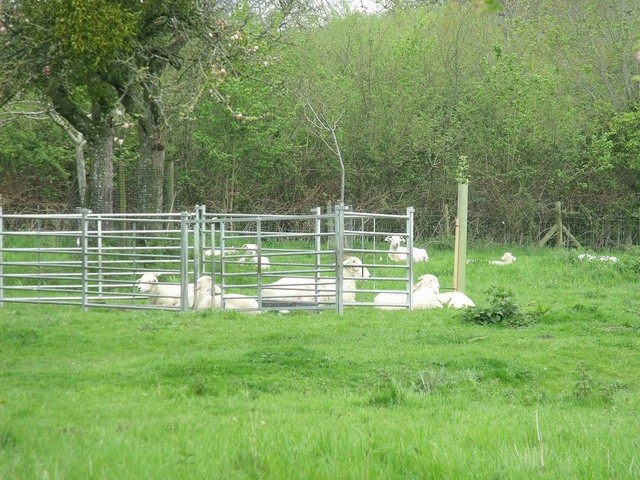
[374,274,443,311]
[383,236,430,265]
[194,276,260,310]
[489,252,518,266]
[411,282,477,310]
[203,244,239,258]
[238,244,271,271]
[260,255,371,314]
[136,272,199,312]
